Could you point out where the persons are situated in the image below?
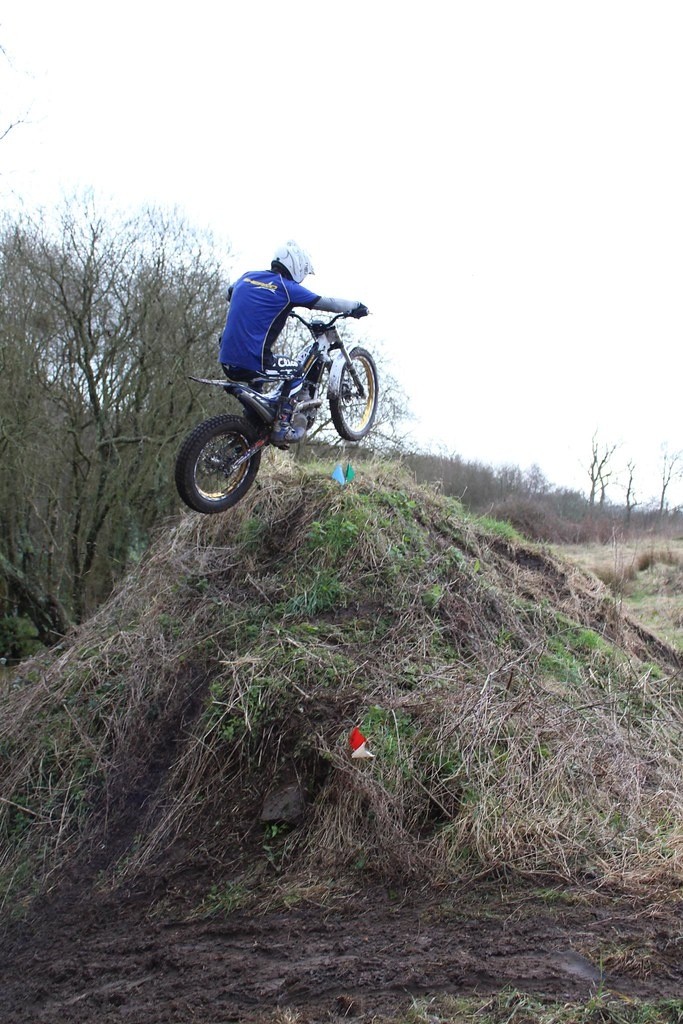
[217,239,369,446]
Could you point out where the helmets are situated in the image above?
[271,244,310,284]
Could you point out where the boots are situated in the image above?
[270,398,307,445]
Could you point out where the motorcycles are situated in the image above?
[174,306,380,515]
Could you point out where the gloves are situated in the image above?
[351,304,367,319]
